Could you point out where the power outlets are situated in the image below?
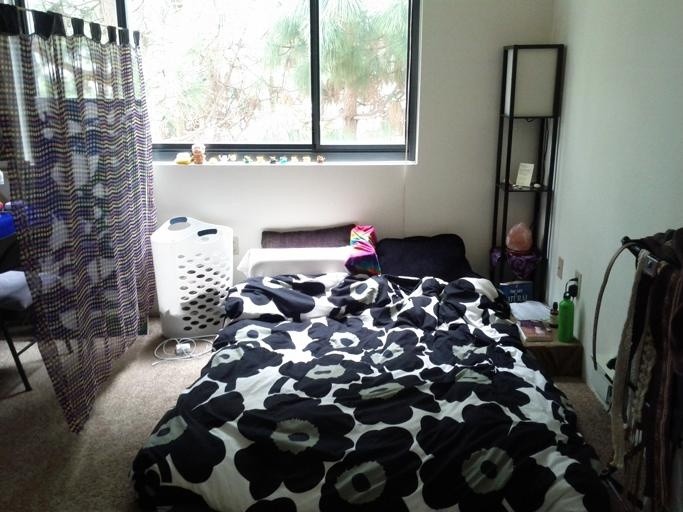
[573,270,582,299]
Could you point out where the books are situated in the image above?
[517,317,553,342]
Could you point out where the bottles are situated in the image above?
[557,292,574,342]
[192,144,205,164]
[549,302,559,327]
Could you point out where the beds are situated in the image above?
[145,239,620,511]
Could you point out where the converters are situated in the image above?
[175,343,190,354]
[568,285,578,297]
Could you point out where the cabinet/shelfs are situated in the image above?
[492,43,565,301]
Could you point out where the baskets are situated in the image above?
[149,216,233,342]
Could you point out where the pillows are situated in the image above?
[246,222,383,281]
